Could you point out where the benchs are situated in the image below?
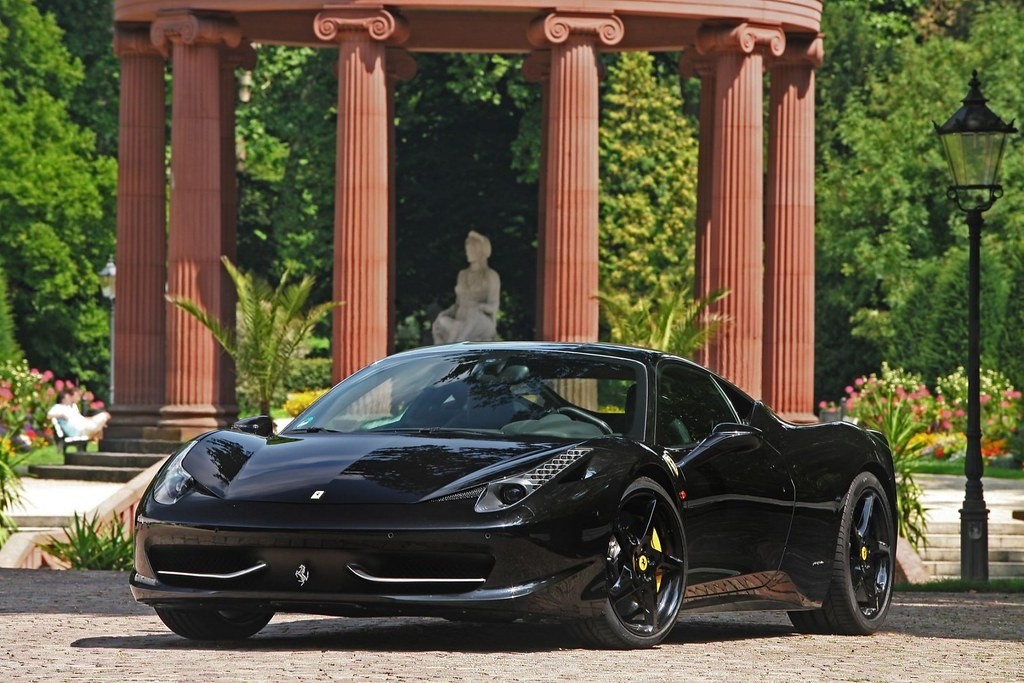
[51,416,89,459]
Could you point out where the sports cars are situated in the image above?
[129,341,898,648]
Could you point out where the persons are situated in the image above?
[433,231,500,345]
[46,389,110,442]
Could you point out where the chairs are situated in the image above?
[622,384,688,448]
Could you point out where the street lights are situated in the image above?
[932,68,1018,586]
[98,254,116,404]
